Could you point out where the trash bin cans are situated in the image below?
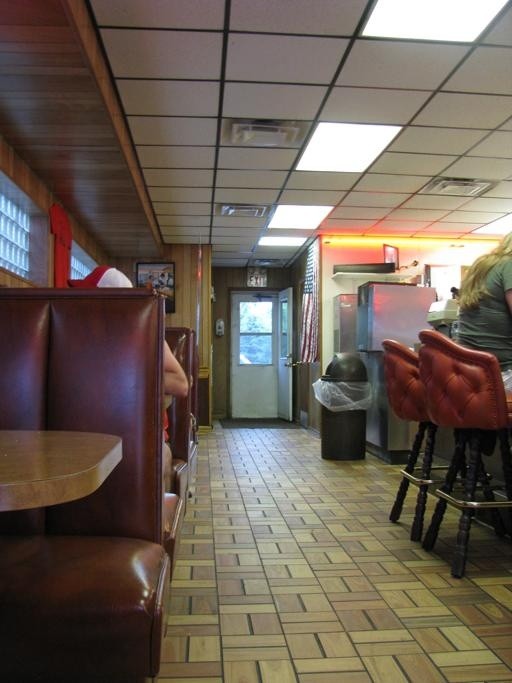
[321,353,369,460]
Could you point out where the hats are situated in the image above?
[68,265,132,288]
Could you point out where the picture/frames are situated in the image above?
[136,262,175,313]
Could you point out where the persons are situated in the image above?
[157,270,166,287]
[456,228,512,394]
[146,274,155,289]
[64,261,191,451]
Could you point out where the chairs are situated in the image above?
[381,328,512,577]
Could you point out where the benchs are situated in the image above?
[0,286,199,683]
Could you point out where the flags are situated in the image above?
[297,242,319,369]
